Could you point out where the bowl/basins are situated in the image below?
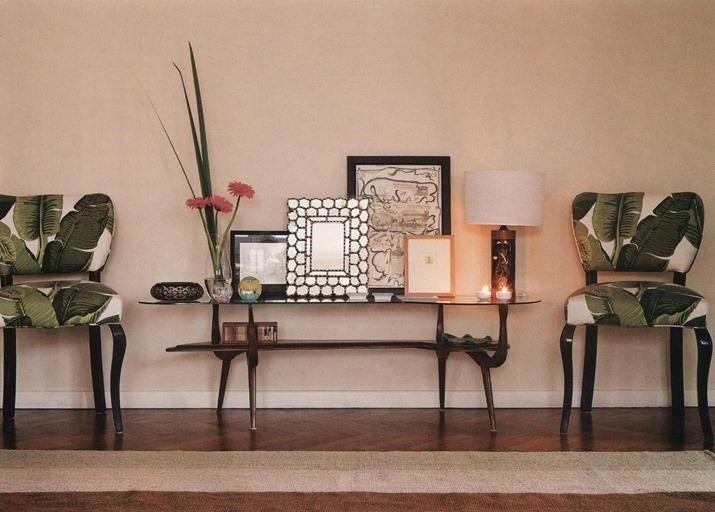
[496,292,514,301]
[477,291,491,300]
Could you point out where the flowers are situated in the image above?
[128,39,256,293]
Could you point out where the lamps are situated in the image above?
[464,170,546,303]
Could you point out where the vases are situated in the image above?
[202,233,234,304]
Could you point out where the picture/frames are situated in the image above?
[286,198,370,300]
[347,154,452,294]
[402,235,457,298]
[228,229,288,298]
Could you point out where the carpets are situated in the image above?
[0,446,715,512]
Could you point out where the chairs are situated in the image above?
[0,193,127,434]
[561,191,715,447]
